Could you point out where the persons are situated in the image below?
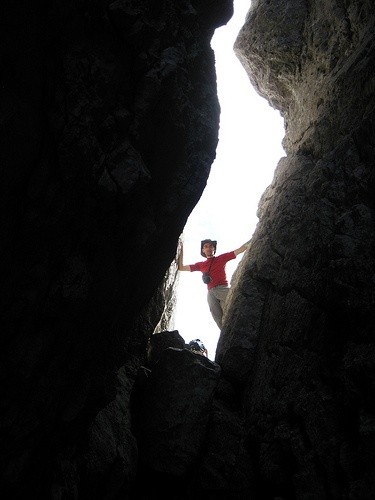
[177,237,252,331]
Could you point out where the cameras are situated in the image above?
[201,272,213,284]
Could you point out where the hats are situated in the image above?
[201,239,217,257]
